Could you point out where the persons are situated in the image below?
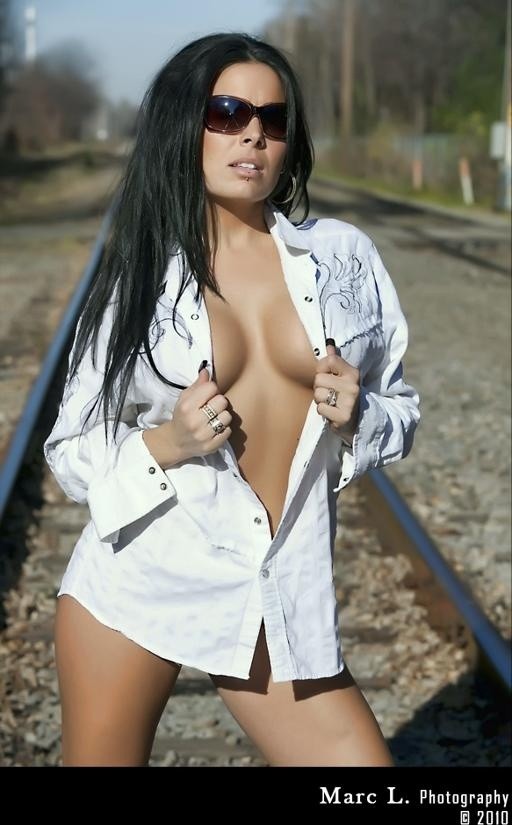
[44,35,420,767]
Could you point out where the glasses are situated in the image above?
[204,95,287,142]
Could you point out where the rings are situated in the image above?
[326,390,338,407]
[202,404,219,423]
[210,417,226,434]
